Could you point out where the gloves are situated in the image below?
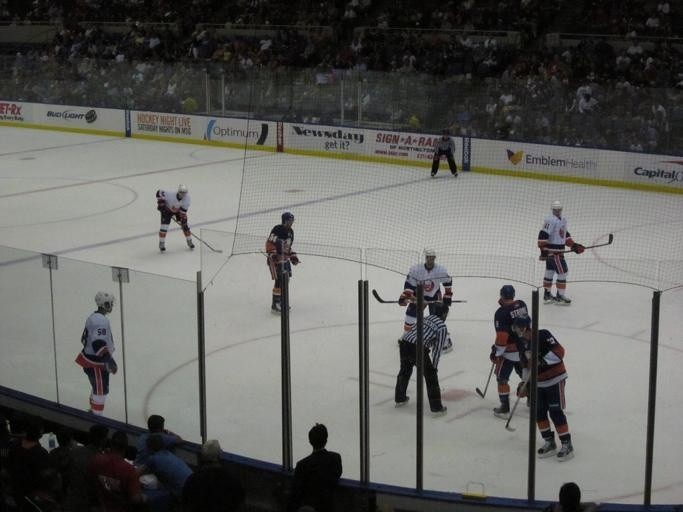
[104,356,118,375]
[399,291,411,307]
[290,252,299,265]
[516,382,528,397]
[490,353,503,366]
[571,243,585,254]
[443,292,453,306]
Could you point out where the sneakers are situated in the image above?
[537,432,557,454]
[443,338,453,350]
[493,404,510,413]
[430,406,447,413]
[271,303,281,314]
[543,289,555,301]
[454,173,458,177]
[556,437,573,457]
[431,172,435,176]
[187,239,195,249]
[288,305,292,311]
[159,241,166,251]
[555,291,571,303]
[395,396,409,403]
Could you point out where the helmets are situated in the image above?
[281,212,294,225]
[512,314,531,326]
[551,200,562,210]
[500,285,516,299]
[433,304,449,314]
[423,246,436,257]
[442,128,449,135]
[177,184,189,193]
[95,290,115,309]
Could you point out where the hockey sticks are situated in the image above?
[561,233,614,253]
[504,368,532,431]
[171,217,222,253]
[475,362,494,399]
[372,289,467,305]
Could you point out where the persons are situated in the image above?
[487,285,529,420]
[398,248,454,352]
[0,0,274,125]
[513,314,575,461]
[547,482,595,512]
[266,212,301,314]
[291,424,342,511]
[0,415,222,512]
[73,289,118,416]
[394,303,451,415]
[275,0,459,134]
[155,184,195,251]
[460,0,683,157]
[537,201,584,306]
[430,128,458,177]
